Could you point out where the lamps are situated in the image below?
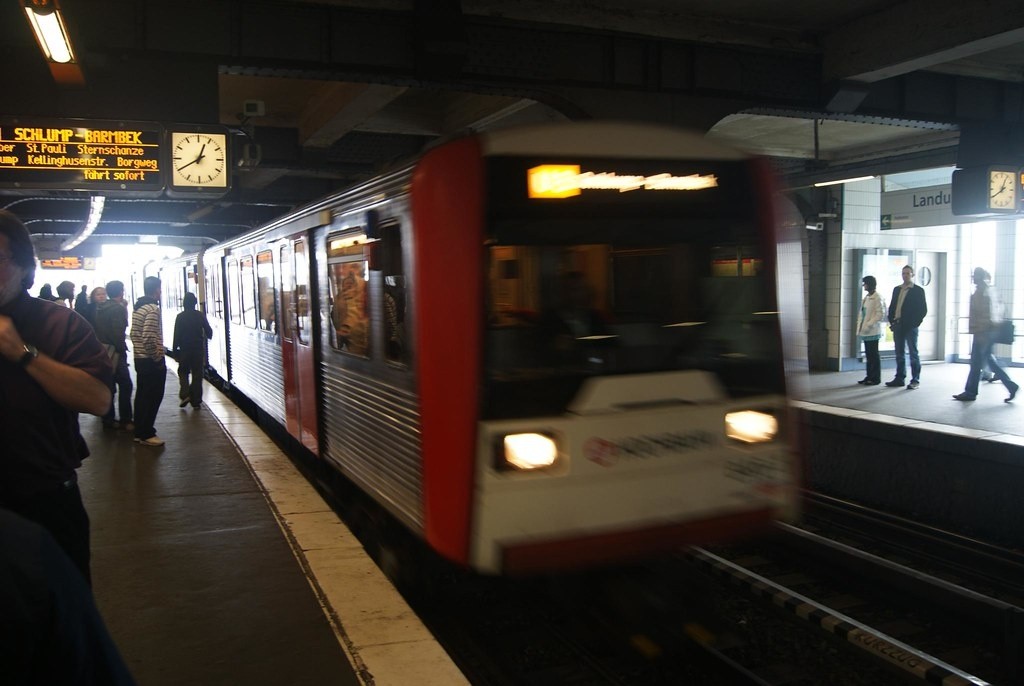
[19,0,88,84]
[814,175,879,187]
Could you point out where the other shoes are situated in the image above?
[125,422,134,432]
[953,392,976,401]
[1005,386,1018,402]
[111,420,119,430]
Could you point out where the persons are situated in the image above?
[257,263,399,356]
[36,280,135,431]
[855,275,885,386]
[884,265,928,390]
[172,292,212,411]
[498,269,623,368]
[132,275,167,446]
[0,206,117,604]
[952,265,1019,403]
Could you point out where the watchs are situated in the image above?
[16,341,40,368]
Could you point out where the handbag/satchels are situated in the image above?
[992,320,1014,345]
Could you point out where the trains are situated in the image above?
[125,119,803,581]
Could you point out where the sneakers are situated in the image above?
[133,436,165,447]
[193,406,200,411]
[885,376,905,387]
[906,380,919,389]
[180,397,191,409]
[858,376,880,386]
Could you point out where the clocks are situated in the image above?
[952,164,1021,218]
[163,123,233,200]
[81,257,97,270]
